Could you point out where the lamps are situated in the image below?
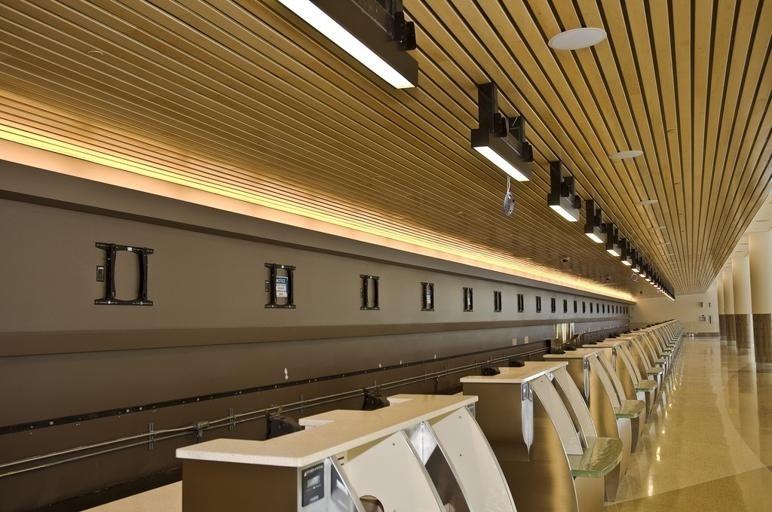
[262,0,416,101]
[544,158,677,303]
[471,77,532,188]
